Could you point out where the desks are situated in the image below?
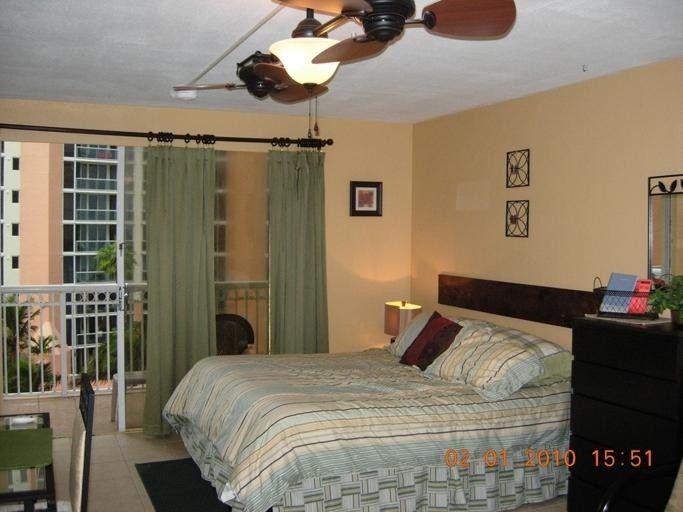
[0,412,57,512]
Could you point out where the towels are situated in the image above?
[0,428,52,469]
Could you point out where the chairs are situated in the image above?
[215,314,256,354]
[1,373,96,512]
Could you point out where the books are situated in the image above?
[599,271,640,314]
[3,414,39,430]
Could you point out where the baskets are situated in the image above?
[593,274,673,320]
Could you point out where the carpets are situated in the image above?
[136,458,232,512]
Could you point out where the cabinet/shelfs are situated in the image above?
[567,317,682,512]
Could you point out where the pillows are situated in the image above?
[424,321,544,401]
[385,312,487,368]
[399,310,463,371]
[479,322,572,388]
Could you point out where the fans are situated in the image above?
[272,0,516,66]
[172,51,329,105]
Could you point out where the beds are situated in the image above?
[162,310,572,512]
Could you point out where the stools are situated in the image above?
[112,369,146,422]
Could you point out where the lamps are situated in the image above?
[383,298,422,342]
[269,10,346,139]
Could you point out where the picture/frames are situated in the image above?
[350,180,383,217]
[505,200,529,238]
[506,148,530,188]
